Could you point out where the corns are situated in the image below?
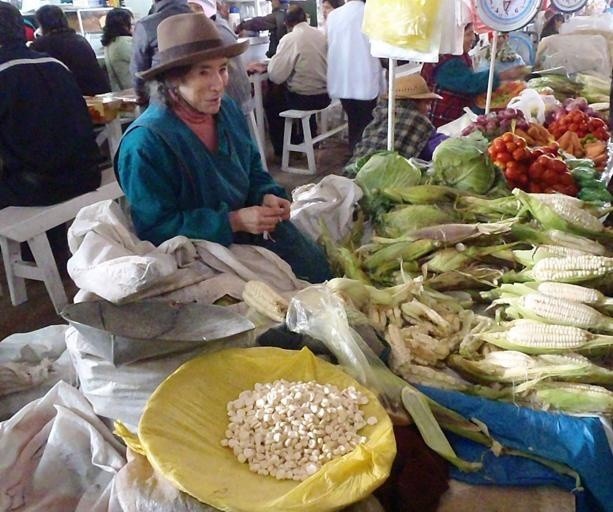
[323,183,498,399]
[242,280,289,321]
[451,184,613,412]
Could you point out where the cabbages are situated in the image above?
[355,149,422,189]
[431,130,495,193]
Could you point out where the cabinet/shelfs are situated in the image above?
[217,0,273,46]
[21,6,131,59]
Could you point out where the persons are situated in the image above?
[24,4,109,101]
[320,1,345,20]
[259,3,333,166]
[234,0,292,59]
[335,72,445,183]
[127,0,254,117]
[324,0,386,155]
[98,7,140,97]
[415,22,533,131]
[0,0,104,294]
[537,13,565,40]
[111,7,337,286]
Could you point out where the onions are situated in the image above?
[461,108,529,135]
[550,97,602,120]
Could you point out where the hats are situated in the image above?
[380,73,444,101]
[135,12,249,80]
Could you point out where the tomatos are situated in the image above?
[489,110,610,196]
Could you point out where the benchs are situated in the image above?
[0,168,128,318]
[279,40,488,176]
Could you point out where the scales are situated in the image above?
[470,0,587,116]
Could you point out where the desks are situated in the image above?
[243,58,277,175]
[80,87,268,169]
